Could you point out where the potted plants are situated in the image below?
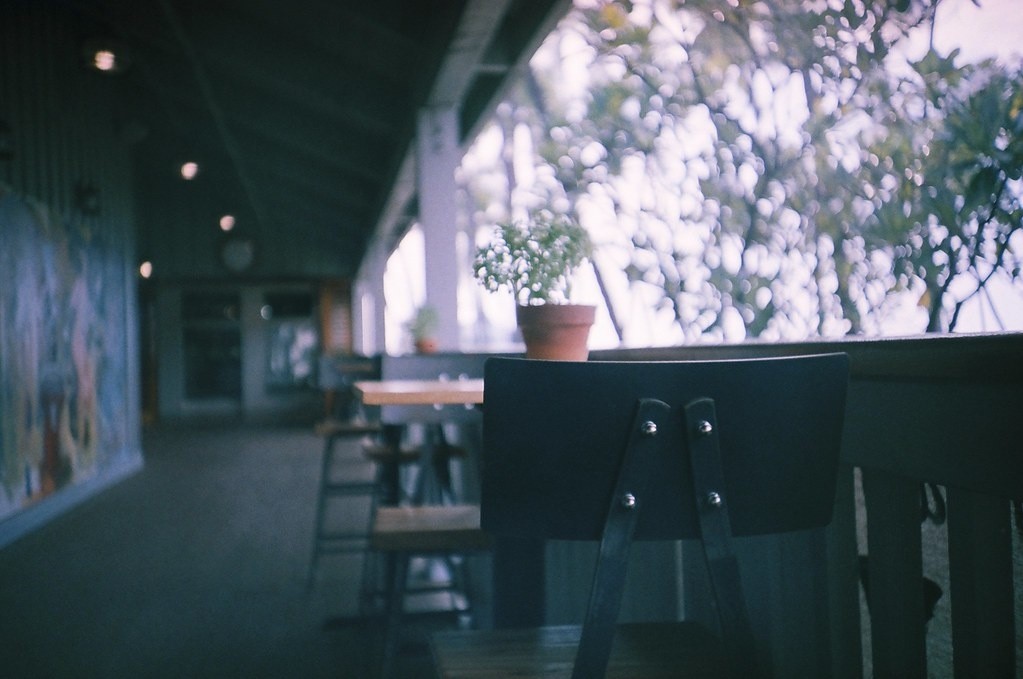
[403,303,439,355]
[472,208,597,363]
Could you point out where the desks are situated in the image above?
[350,379,550,629]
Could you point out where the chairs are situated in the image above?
[426,351,849,679]
[301,352,485,679]
